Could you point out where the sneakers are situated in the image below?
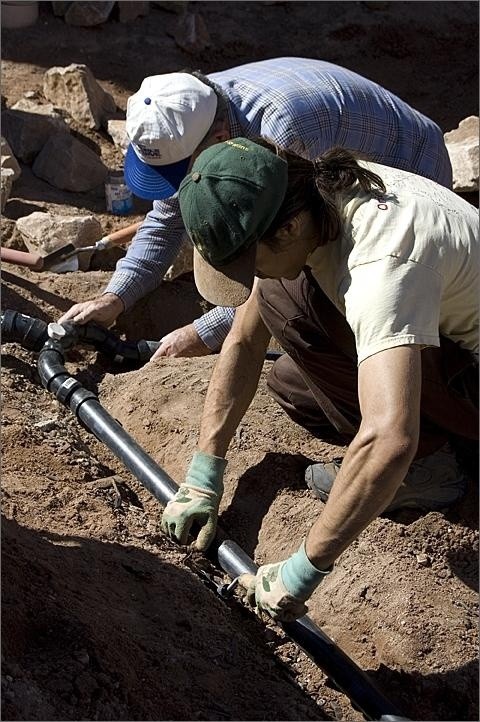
[306,460,461,512]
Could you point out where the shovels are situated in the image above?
[41,219,144,275]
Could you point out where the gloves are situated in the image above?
[245,534,333,625]
[160,448,230,553]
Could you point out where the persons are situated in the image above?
[56,52,452,361]
[159,131,479,623]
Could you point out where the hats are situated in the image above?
[123,71,218,201]
[177,136,289,308]
